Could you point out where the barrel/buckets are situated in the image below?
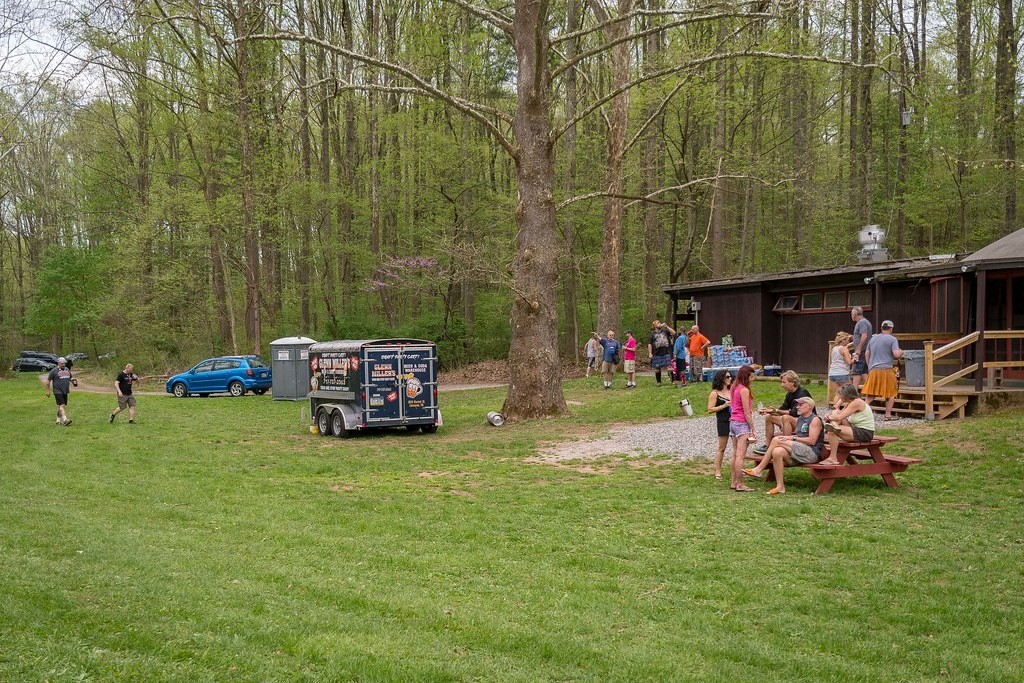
[312,426,318,433]
[487,411,504,426]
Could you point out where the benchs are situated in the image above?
[825,445,922,465]
[744,455,843,469]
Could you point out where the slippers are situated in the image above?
[824,423,841,434]
[769,489,786,495]
[819,458,839,464]
[715,474,722,479]
[730,486,737,489]
[735,487,755,491]
[741,469,763,478]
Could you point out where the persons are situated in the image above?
[861,320,904,421]
[622,330,637,389]
[741,397,824,495]
[707,369,734,479]
[848,306,872,391]
[591,330,621,390]
[753,370,817,454]
[819,383,875,465]
[583,337,596,378]
[829,332,854,409]
[648,320,711,387]
[45,357,77,426]
[109,364,140,424]
[729,365,757,492]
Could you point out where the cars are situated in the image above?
[65,352,87,366]
[13,358,57,372]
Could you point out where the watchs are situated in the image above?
[793,436,796,441]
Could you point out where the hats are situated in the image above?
[881,320,894,327]
[624,329,632,334]
[57,357,67,363]
[794,397,816,407]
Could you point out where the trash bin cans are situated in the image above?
[902,350,925,387]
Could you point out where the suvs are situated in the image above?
[21,350,73,370]
[166,354,272,398]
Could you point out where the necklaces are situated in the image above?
[721,389,730,400]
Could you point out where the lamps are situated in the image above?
[864,277,875,284]
[961,266,972,272]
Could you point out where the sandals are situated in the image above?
[884,416,899,421]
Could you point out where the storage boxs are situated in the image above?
[764,365,781,376]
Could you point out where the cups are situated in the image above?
[72,379,78,387]
[687,330,692,335]
[747,437,755,443]
[757,401,763,412]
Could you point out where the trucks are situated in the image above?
[307,337,443,439]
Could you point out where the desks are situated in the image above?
[755,433,908,495]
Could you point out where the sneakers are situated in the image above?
[754,445,767,451]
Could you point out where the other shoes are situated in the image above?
[831,405,840,410]
[109,414,115,424]
[691,379,703,384]
[129,420,137,424]
[625,385,636,388]
[56,422,62,425]
[64,419,72,426]
[603,385,611,389]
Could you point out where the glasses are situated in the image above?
[725,375,732,379]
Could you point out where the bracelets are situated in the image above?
[775,409,778,413]
[626,348,627,350]
[720,405,723,410]
